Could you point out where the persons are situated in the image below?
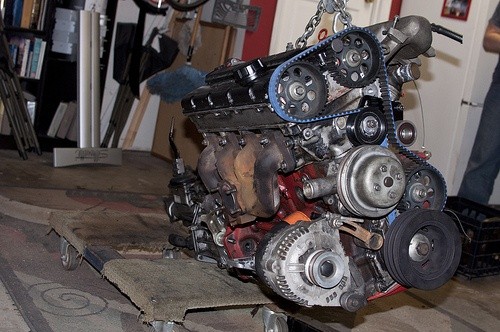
[455,0,500,224]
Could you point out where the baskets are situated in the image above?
[444,195,500,280]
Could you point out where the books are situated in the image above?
[0,0,48,31]
[7,33,46,79]
[66,108,80,142]
[57,99,78,139]
[46,101,68,138]
[0,89,36,136]
